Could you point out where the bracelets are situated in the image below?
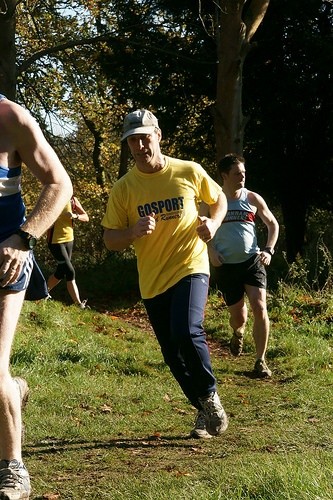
[76,213,79,219]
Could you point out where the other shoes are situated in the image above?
[230,330,244,356]
[11,376,30,415]
[254,359,272,377]
[190,411,213,438]
[197,392,229,437]
[75,303,86,309]
[0,459,31,500]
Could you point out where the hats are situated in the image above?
[120,108,160,141]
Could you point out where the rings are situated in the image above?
[203,236,204,239]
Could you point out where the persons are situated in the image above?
[0,92,73,500]
[101,110,229,439]
[36,196,91,310]
[198,154,279,377]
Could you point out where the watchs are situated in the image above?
[16,229,37,250]
[264,247,275,255]
